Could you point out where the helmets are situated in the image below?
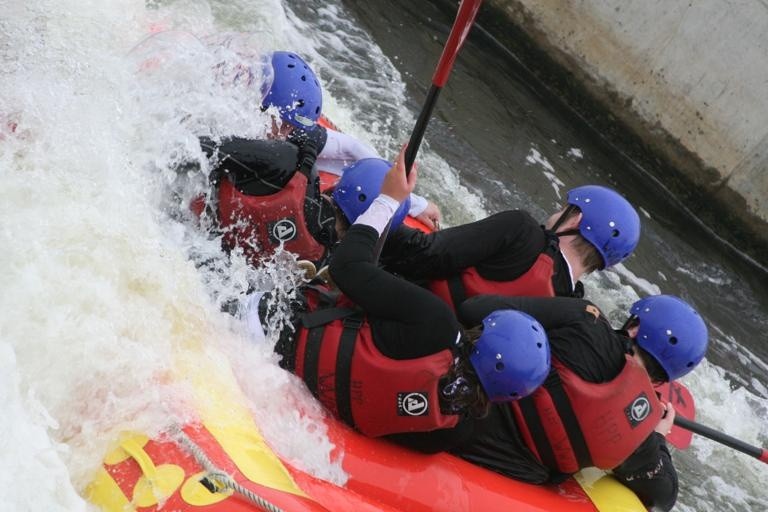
[468,308,553,404]
[333,157,412,238]
[256,51,323,134]
[565,185,643,271]
[629,294,709,383]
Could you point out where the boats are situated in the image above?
[53,20,697,511]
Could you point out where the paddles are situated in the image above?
[405,0,478,178]
